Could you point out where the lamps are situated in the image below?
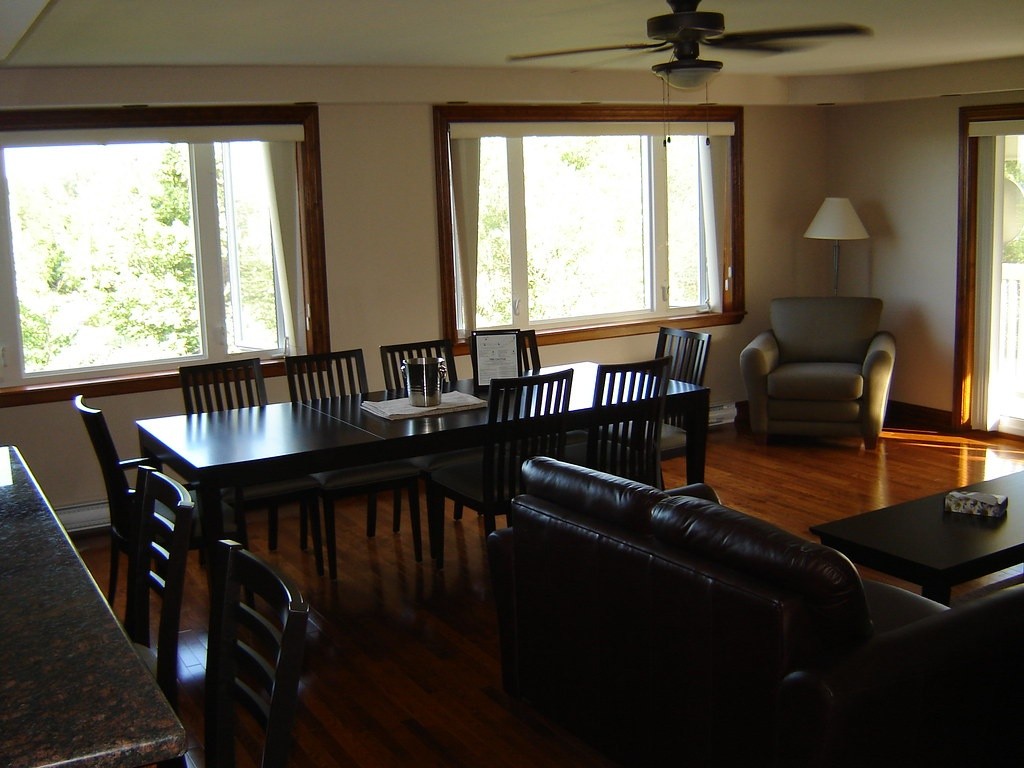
[802,197,870,297]
[651,59,724,89]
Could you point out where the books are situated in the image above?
[360,390,488,420]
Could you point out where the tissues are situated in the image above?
[944,490,1008,518]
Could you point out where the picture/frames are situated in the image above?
[473,329,518,392]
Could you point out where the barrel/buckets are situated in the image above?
[401,358,447,407]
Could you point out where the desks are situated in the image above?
[0,443,190,768]
[135,359,738,599]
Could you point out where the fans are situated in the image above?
[505,0,879,61]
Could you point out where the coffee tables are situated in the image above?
[807,470,1024,607]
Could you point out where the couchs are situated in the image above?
[487,456,1024,768]
[739,297,896,450]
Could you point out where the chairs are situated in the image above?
[598,325,713,489]
[540,353,673,491]
[424,367,576,565]
[378,336,466,534]
[469,328,541,371]
[75,395,251,613]
[178,358,326,577]
[203,537,309,768]
[283,348,424,579]
[127,465,195,706]
